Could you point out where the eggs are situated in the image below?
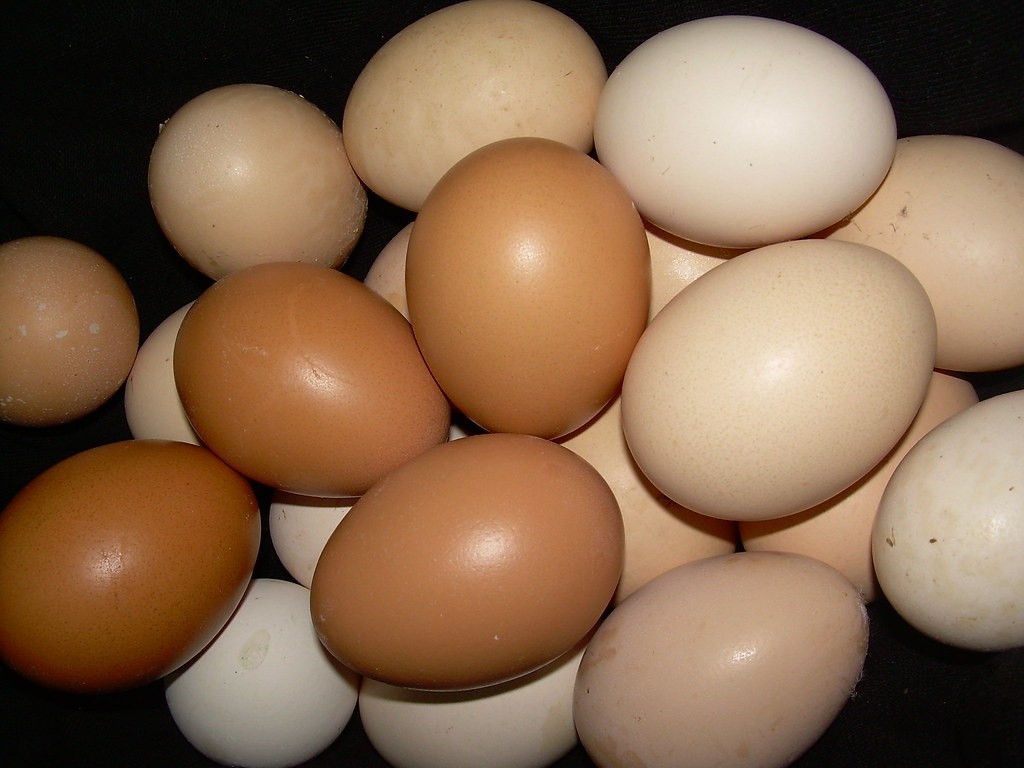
[0,0,1024,768]
